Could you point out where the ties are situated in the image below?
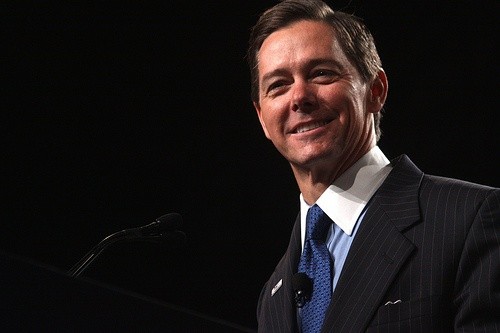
[298,206,334,332]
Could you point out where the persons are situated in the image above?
[245,0,499,333]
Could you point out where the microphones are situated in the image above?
[66,212,186,280]
[291,272,308,306]
